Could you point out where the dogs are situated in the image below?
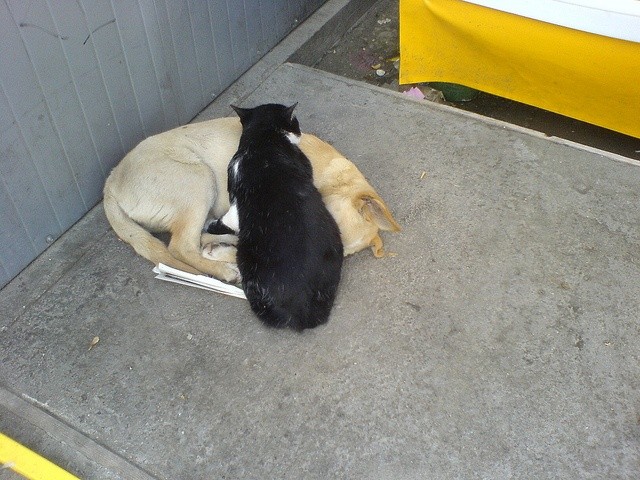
[102,117,402,282]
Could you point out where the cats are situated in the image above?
[206,101,343,333]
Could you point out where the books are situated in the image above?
[152,219,247,300]
